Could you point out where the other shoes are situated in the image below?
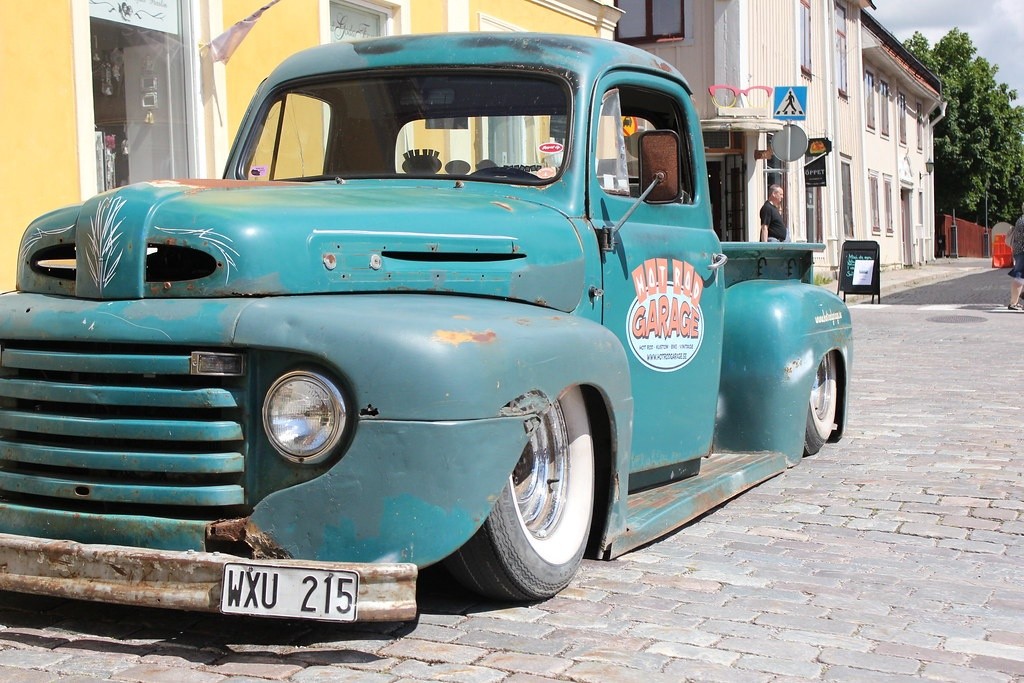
[1007,304,1022,310]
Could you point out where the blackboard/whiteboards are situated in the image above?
[837,241,880,294]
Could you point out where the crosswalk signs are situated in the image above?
[774,85,807,121]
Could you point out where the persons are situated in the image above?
[1007,203,1024,310]
[760,184,786,242]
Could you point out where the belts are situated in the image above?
[768,234,783,242]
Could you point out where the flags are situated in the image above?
[206,0,280,65]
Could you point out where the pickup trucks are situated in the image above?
[0,30,853,623]
